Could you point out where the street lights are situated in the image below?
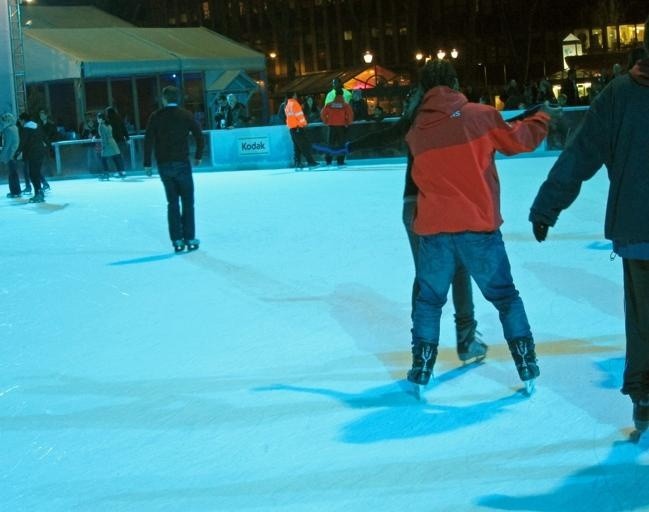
[476,62,488,88]
[362,44,379,107]
[414,47,460,62]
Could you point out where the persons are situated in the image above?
[142,85,207,247]
[213,75,369,167]
[468,71,579,107]
[526,21,649,426]
[388,86,489,361]
[403,56,565,386]
[1,104,130,204]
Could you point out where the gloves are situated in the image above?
[531,221,550,244]
[541,100,563,126]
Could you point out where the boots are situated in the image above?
[306,161,321,167]
[7,192,19,196]
[457,340,489,361]
[632,400,649,432]
[173,239,185,248]
[29,189,45,201]
[293,159,307,168]
[185,239,201,246]
[97,170,126,180]
[507,335,540,382]
[405,341,438,385]
